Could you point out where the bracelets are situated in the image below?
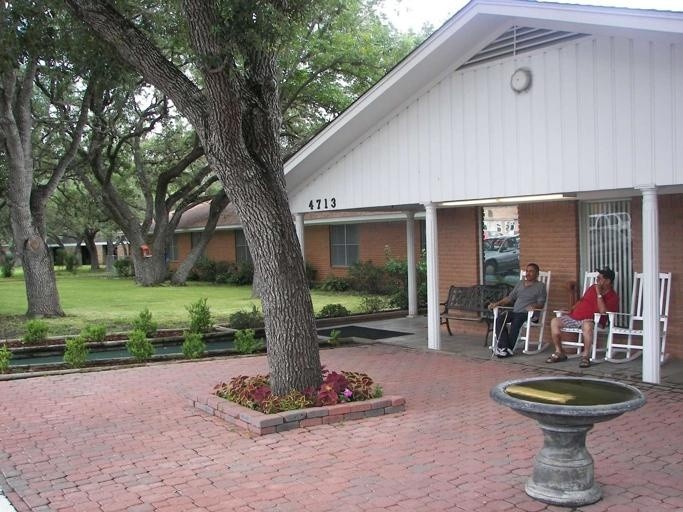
[597,294,603,298]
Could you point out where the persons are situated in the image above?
[546,266,619,368]
[488,263,547,356]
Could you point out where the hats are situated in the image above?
[595,266,616,282]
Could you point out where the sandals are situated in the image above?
[546,352,567,363]
[578,356,591,367]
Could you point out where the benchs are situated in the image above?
[437,285,514,348]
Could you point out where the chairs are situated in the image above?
[489,269,551,356]
[554,271,619,364]
[605,271,672,369]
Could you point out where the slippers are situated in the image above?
[495,347,514,357]
